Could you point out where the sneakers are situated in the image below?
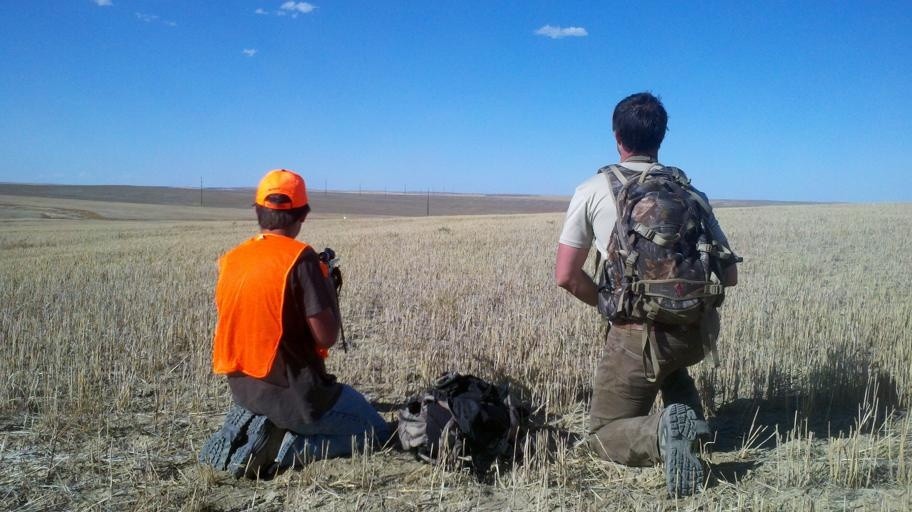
[690,419,713,453]
[657,403,704,500]
[227,414,281,479]
[200,404,255,471]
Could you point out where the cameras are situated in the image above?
[319,248,343,288]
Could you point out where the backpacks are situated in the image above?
[593,164,742,330]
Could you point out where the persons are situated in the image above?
[202,167,391,480]
[551,92,742,500]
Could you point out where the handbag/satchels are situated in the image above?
[397,370,532,484]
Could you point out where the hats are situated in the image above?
[255,169,307,210]
